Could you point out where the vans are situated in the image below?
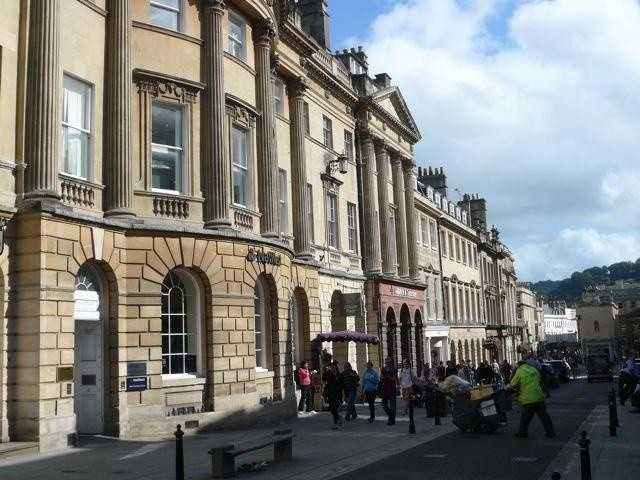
[588,357,614,382]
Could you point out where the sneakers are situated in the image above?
[345,415,357,421]
[338,415,343,426]
[331,424,338,429]
[297,410,318,415]
[545,432,555,439]
[368,416,375,424]
[514,432,528,438]
[377,379,392,400]
[402,409,408,415]
[412,383,421,393]
[387,415,395,425]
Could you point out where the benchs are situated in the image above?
[207,429,296,479]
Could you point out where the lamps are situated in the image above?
[325,151,349,175]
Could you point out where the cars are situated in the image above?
[547,360,572,383]
[539,364,560,390]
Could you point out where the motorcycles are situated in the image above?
[447,378,499,435]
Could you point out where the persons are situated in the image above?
[297,361,318,414]
[321,350,583,430]
[619,351,640,406]
[504,360,556,438]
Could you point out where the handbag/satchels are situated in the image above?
[322,384,329,397]
[542,384,550,399]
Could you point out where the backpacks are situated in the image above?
[293,370,300,385]
